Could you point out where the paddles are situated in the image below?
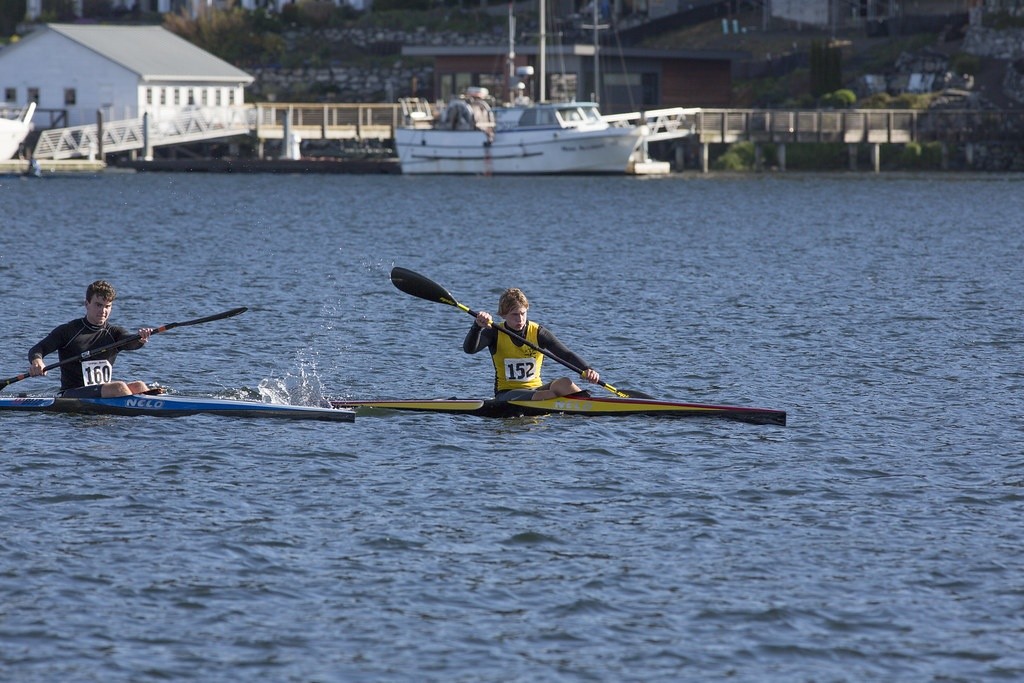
[0,307,248,389]
[389,265,654,399]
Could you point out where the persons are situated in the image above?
[462,288,600,402]
[29,279,155,398]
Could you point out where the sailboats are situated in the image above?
[392,0,674,177]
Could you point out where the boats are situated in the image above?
[321,395,790,430]
[2,392,358,425]
[1,100,108,176]
